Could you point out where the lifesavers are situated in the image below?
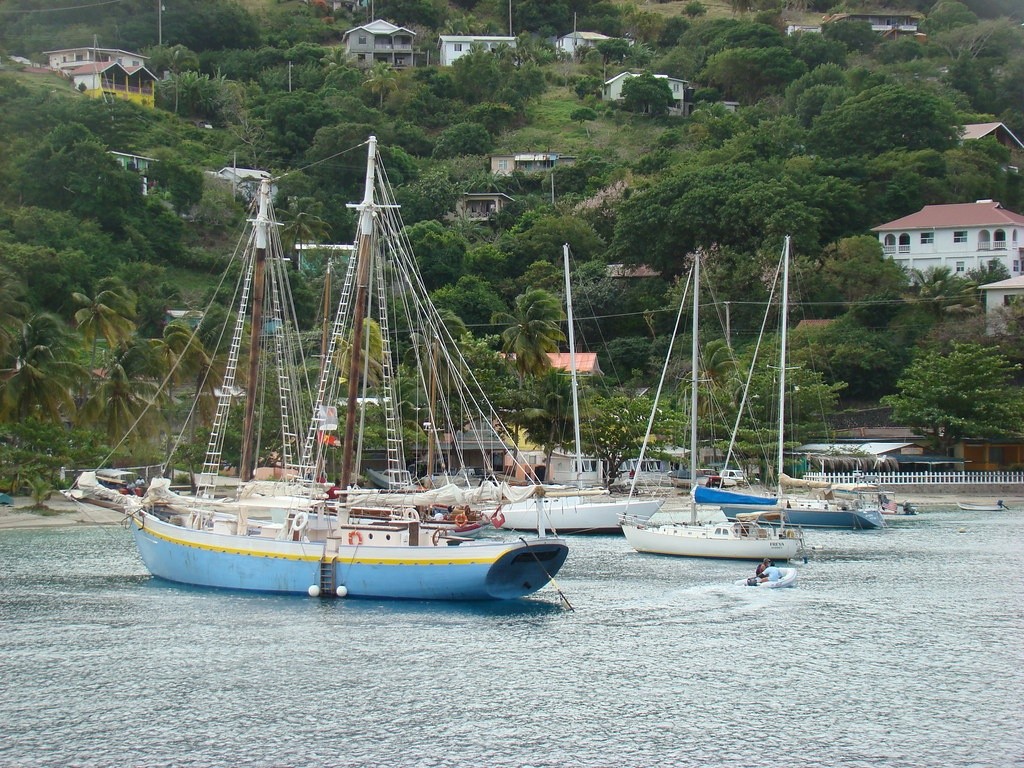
[404,508,420,522]
[292,512,309,531]
[787,530,794,538]
[348,530,363,546]
[455,513,468,526]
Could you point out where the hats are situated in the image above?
[764,558,771,563]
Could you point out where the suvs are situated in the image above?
[695,469,725,487]
[720,470,759,488]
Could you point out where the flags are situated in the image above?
[490,505,505,528]
[318,405,338,431]
[317,432,341,449]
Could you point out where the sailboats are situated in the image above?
[59,136,919,608]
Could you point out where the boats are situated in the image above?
[956,499,1010,512]
[734,566,798,591]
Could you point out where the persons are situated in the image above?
[756,558,782,583]
[127,476,145,490]
[629,469,635,478]
[316,472,326,483]
[326,478,341,500]
